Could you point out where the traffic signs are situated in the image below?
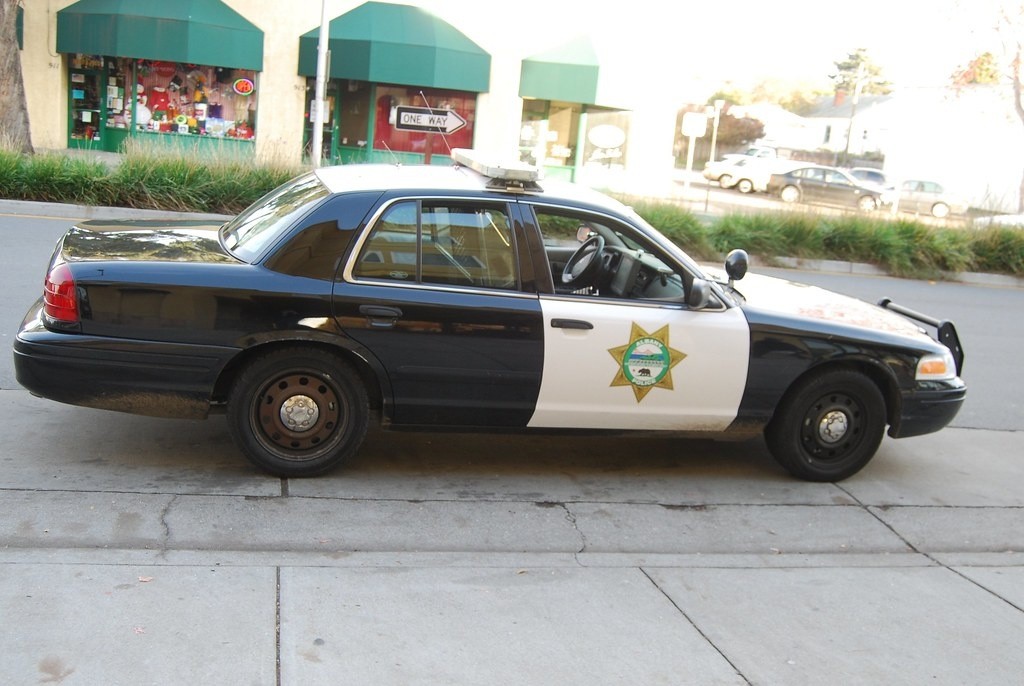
[393,105,467,136]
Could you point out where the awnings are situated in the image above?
[520,48,600,105]
[55,0,265,71]
[297,1,492,95]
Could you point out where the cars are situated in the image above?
[13,148,968,482]
[705,147,968,219]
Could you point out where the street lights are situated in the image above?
[705,99,725,211]
